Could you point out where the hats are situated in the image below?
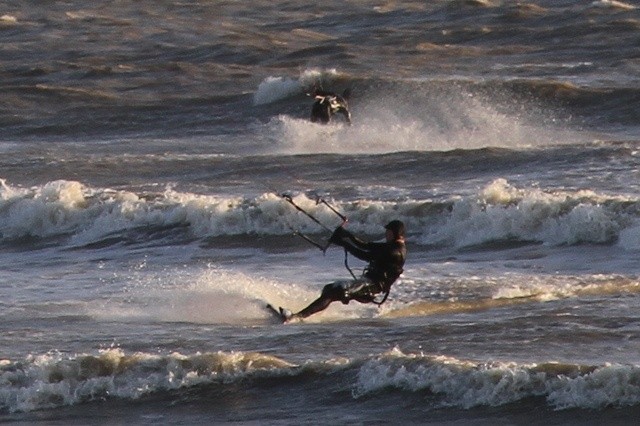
[385,220,406,236]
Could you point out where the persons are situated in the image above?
[261,193,408,321]
[310,89,354,128]
